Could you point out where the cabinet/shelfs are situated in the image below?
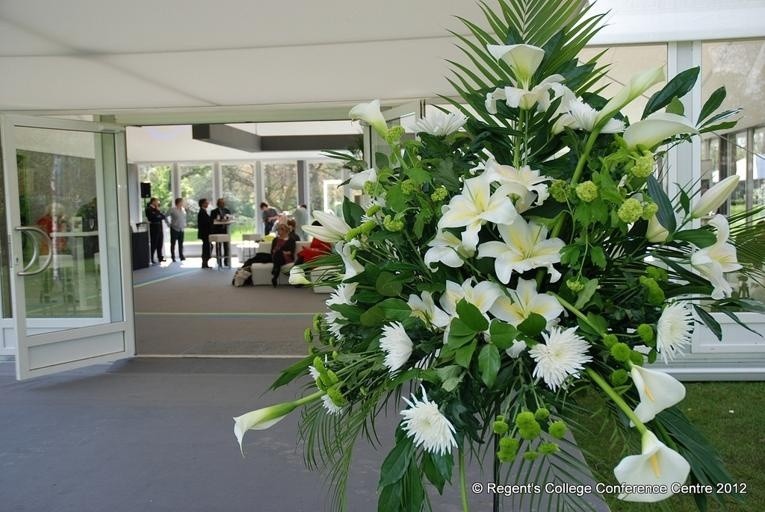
[37,217,98,311]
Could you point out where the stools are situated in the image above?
[209,234,231,271]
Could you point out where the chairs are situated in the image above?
[238,234,261,262]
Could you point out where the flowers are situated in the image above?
[230,0,765,511]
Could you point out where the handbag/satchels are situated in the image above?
[233,266,251,287]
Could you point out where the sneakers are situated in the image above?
[272,276,277,288]
[152,257,185,263]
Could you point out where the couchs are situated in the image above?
[250,242,308,285]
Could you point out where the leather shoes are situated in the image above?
[218,262,228,266]
[201,255,211,269]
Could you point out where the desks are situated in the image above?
[84,231,149,271]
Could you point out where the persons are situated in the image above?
[210,198,231,267]
[164,198,187,262]
[35,203,79,303]
[233,201,331,288]
[145,197,166,263]
[197,198,213,268]
[75,197,101,291]
